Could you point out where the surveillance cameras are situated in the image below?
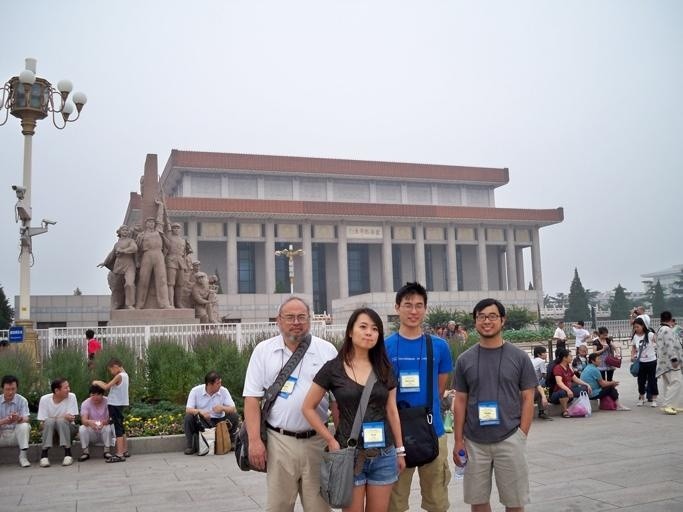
[12,185,26,198]
[42,217,57,225]
[20,226,30,231]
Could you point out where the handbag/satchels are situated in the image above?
[215,419,232,454]
[317,443,355,508]
[197,427,215,456]
[397,407,438,468]
[599,396,617,409]
[603,354,621,368]
[233,419,267,471]
[630,359,639,377]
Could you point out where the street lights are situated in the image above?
[275,242,307,293]
[1,57,85,367]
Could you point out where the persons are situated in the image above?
[91,357,131,463]
[452,298,539,512]
[242,296,338,512]
[381,281,453,512]
[0,375,33,468]
[427,306,682,433]
[80,384,114,462]
[36,378,79,467]
[95,193,221,323]
[183,370,242,455]
[85,328,101,370]
[301,306,408,511]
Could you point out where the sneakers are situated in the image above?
[537,413,553,420]
[635,399,683,414]
[77,453,89,461]
[103,451,130,462]
[183,447,196,454]
[38,456,50,467]
[615,404,630,411]
[18,454,31,467]
[61,455,73,465]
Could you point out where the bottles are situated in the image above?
[453,450,467,480]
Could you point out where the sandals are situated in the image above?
[560,407,570,417]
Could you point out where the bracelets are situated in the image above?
[395,446,405,453]
[397,454,407,456]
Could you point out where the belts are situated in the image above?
[355,444,394,459]
[263,421,327,438]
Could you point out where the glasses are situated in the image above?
[474,313,500,321]
[279,314,310,325]
